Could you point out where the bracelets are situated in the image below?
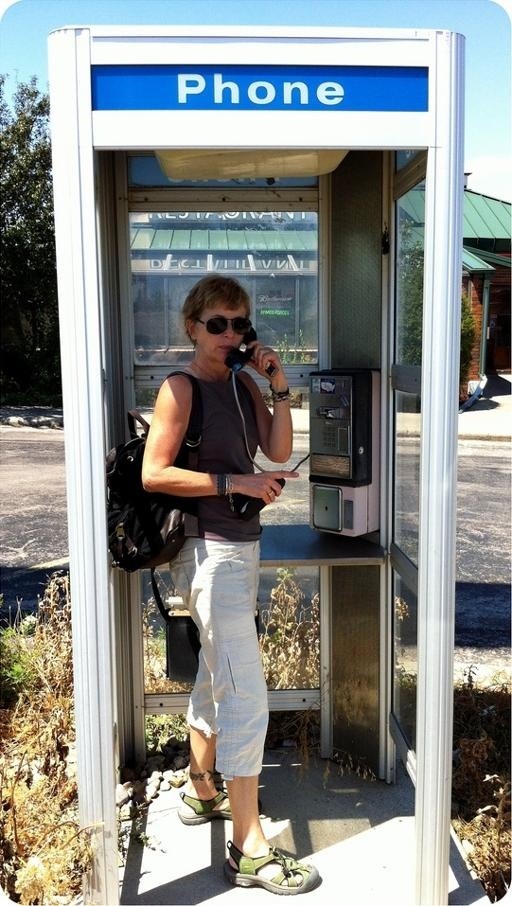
[225,473,235,514]
[270,384,290,402]
[216,473,225,498]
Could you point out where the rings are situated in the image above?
[268,490,274,497]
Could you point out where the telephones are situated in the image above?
[224,327,258,372]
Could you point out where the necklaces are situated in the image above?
[191,362,228,385]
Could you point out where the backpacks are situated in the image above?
[101,369,207,577]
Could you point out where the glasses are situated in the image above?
[189,311,254,337]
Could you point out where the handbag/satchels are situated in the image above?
[164,614,206,685]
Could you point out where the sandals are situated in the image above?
[175,785,264,826]
[222,838,321,896]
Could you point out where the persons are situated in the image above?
[139,273,325,898]
